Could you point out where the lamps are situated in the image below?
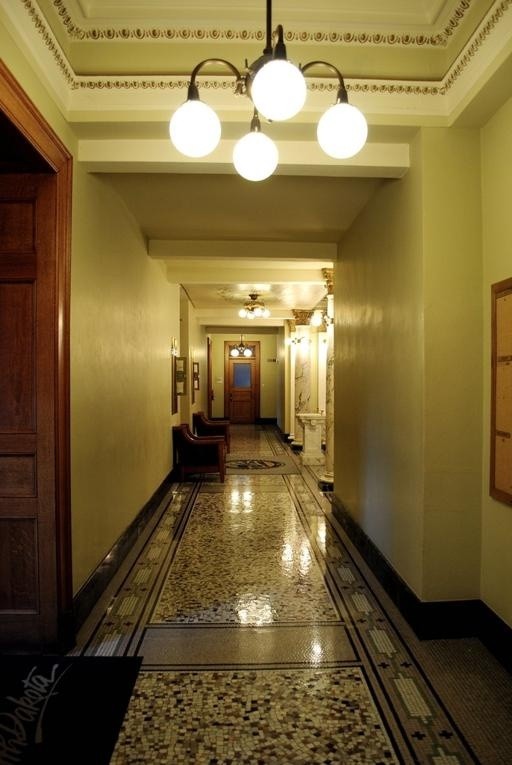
[238,294,271,320]
[169,0,369,182]
[230,335,253,357]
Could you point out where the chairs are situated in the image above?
[194,412,231,453]
[173,424,225,483]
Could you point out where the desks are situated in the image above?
[297,413,326,465]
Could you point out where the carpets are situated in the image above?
[0,656,143,765]
